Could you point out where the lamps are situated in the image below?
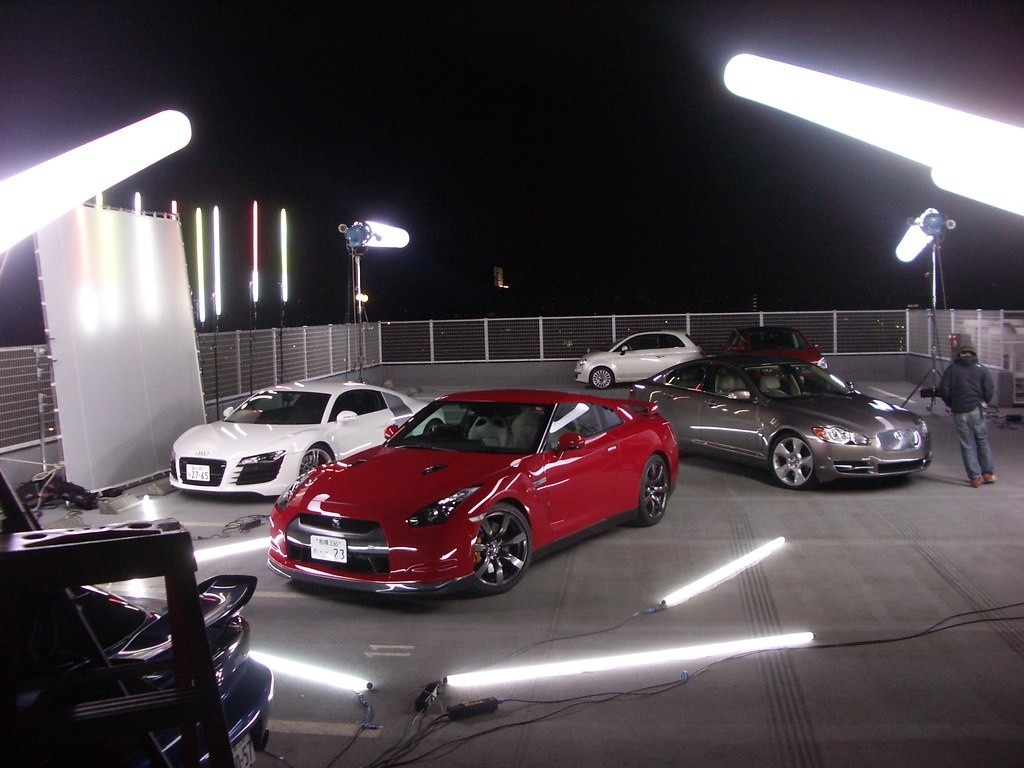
[348,220,409,248]
[444,629,817,686]
[280,208,288,301]
[213,205,222,315]
[723,53,1024,217]
[0,110,192,254]
[253,201,258,303]
[195,207,205,323]
[246,650,371,693]
[658,536,786,607]
[896,206,943,262]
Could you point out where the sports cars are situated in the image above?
[628,353,935,491]
[575,328,708,390]
[168,378,448,497]
[266,388,680,597]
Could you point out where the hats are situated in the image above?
[956,344,977,358]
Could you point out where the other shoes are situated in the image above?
[983,472,997,483]
[971,479,980,487]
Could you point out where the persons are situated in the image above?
[938,344,997,488]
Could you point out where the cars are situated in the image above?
[717,325,831,380]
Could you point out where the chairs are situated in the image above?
[760,375,788,396]
[716,371,735,394]
[469,410,546,448]
